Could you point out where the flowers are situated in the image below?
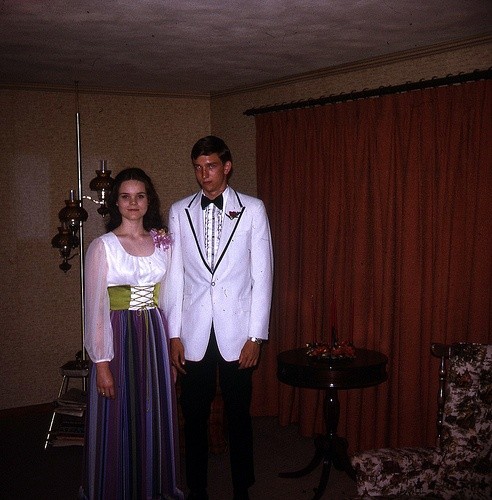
[149,227,175,251]
[225,211,241,220]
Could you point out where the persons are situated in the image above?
[165,136,274,500]
[85,167,184,500]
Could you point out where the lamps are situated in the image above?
[51,160,115,274]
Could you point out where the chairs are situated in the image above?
[351,341,492,500]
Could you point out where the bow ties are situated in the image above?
[201,193,223,210]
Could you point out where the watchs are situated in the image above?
[247,337,263,345]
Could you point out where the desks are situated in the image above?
[275,348,389,500]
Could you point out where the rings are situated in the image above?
[100,392,105,396]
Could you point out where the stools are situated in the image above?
[44,360,90,449]
